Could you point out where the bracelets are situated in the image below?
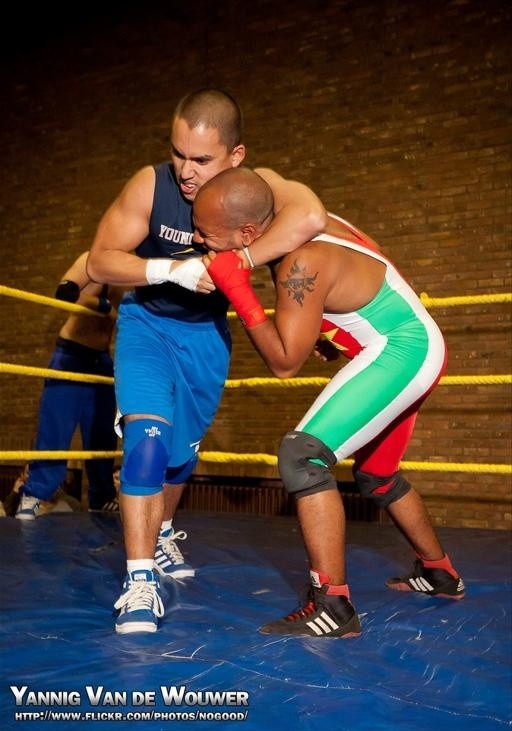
[98,297,113,313]
[145,257,174,286]
[243,245,255,268]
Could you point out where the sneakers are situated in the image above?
[258,567,362,640]
[152,526,196,580]
[87,499,121,513]
[113,569,165,635]
[384,549,466,601]
[15,491,41,521]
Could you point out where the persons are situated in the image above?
[0,462,121,517]
[191,166,466,637]
[14,249,122,520]
[86,89,327,633]
